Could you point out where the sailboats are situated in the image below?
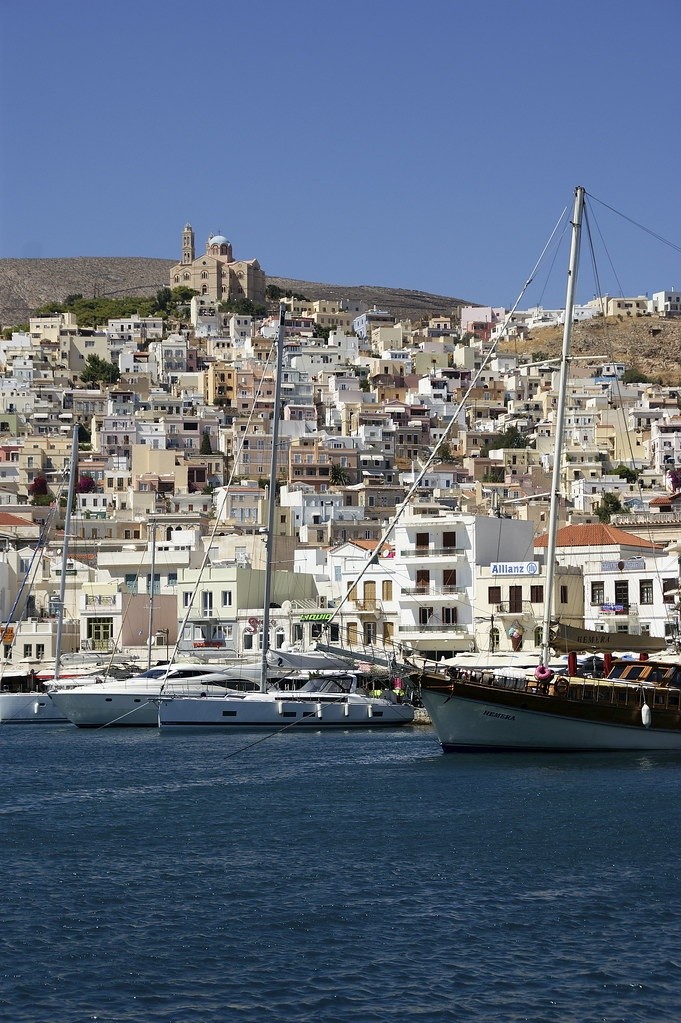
[146,301,414,730]
[312,184,681,756]
[0,421,276,722]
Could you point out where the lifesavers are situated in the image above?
[553,678,569,696]
[535,665,552,680]
[3,685,10,691]
